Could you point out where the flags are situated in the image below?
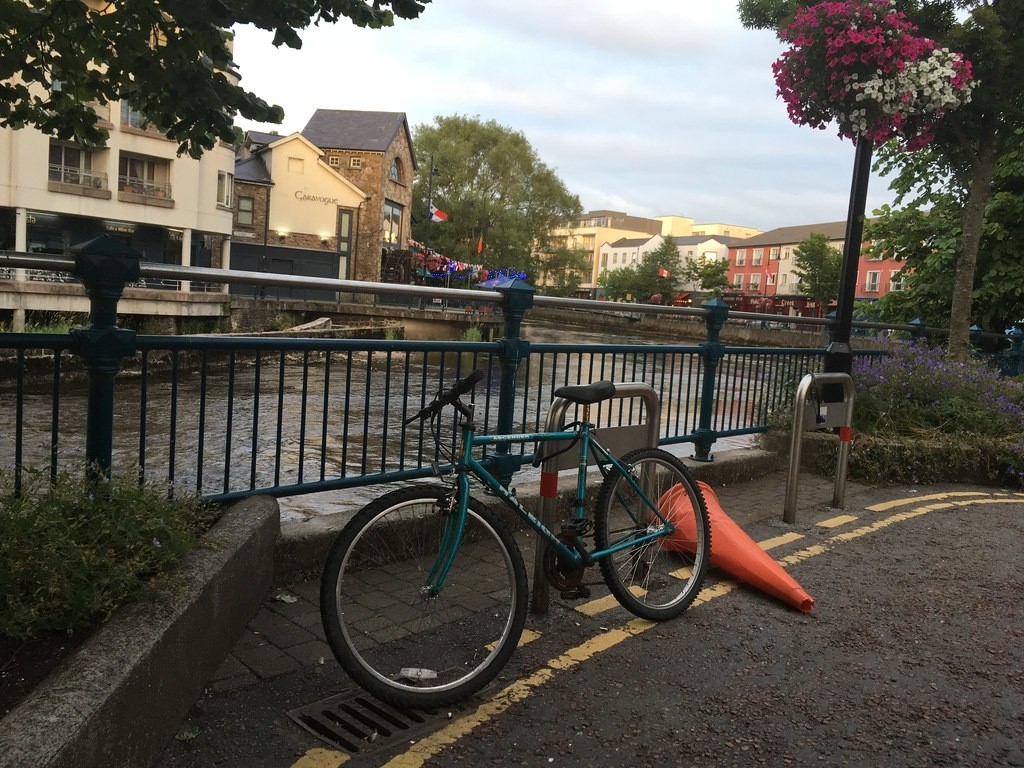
[477,236,483,253]
[429,203,448,223]
[658,267,671,279]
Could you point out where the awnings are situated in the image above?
[672,292,689,299]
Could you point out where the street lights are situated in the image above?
[420,153,442,310]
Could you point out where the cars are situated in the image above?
[745,319,783,331]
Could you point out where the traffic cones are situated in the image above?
[651,479,815,614]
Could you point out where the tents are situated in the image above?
[826,296,885,337]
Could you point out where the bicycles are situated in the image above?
[318,368,713,708]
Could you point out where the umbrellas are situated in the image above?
[478,275,513,305]
